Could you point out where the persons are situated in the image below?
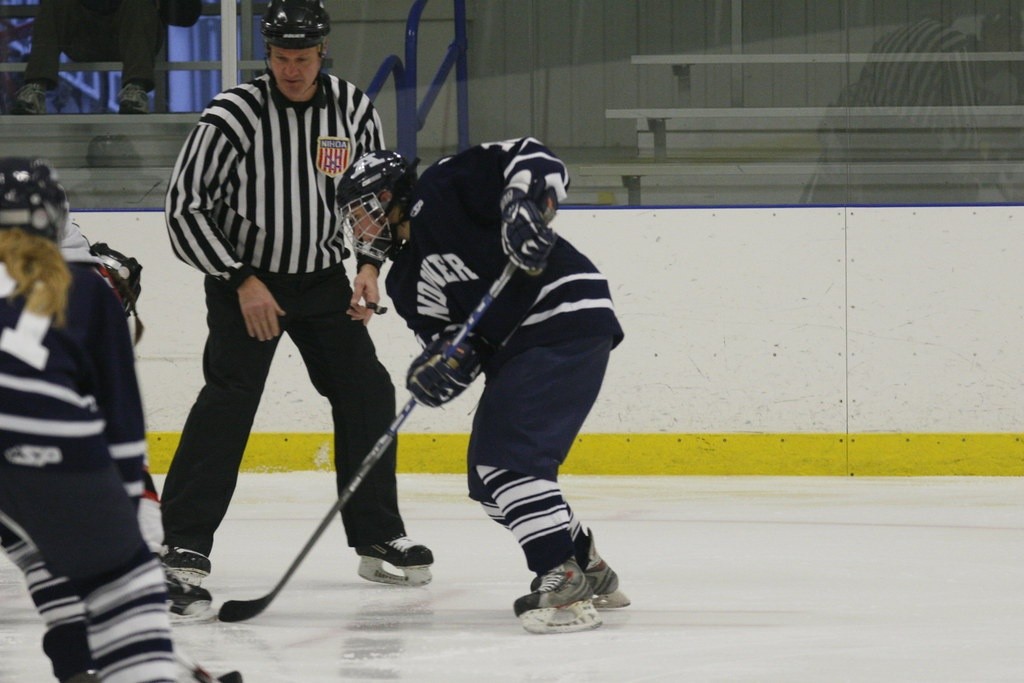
[156,0,434,588]
[797,0,1024,202]
[0,155,245,683]
[17,0,205,112]
[337,134,633,635]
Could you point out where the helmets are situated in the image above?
[260,0,331,48]
[336,150,421,260]
[90,242,143,316]
[0,154,69,246]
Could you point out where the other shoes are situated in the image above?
[117,81,149,113]
[11,80,46,114]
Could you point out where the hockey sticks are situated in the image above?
[216,194,557,625]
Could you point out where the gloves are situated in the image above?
[499,188,558,275]
[407,338,482,408]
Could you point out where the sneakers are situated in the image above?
[356,536,433,586]
[530,527,631,609]
[161,544,212,625]
[514,557,603,633]
